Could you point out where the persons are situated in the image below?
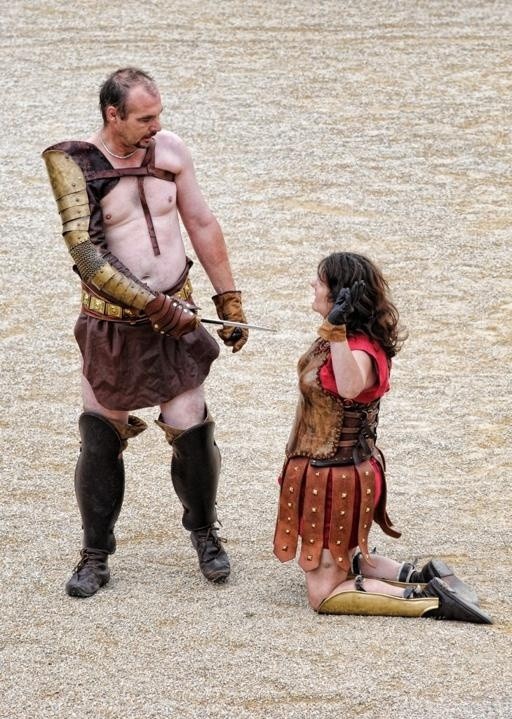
[43,67,251,597]
[272,250,494,626]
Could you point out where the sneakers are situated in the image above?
[66,558,110,598]
[415,559,493,624]
[190,526,231,582]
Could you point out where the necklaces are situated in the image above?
[98,127,142,160]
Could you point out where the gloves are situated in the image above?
[327,280,366,325]
[212,290,249,353]
